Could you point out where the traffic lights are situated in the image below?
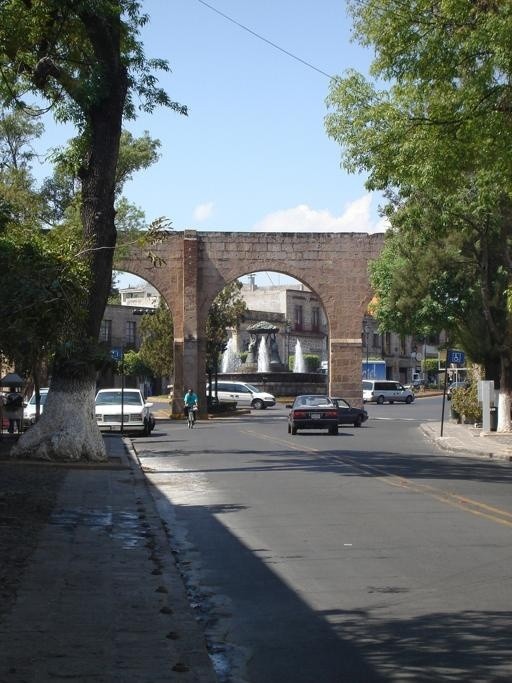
[221,340,227,354]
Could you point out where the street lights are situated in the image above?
[365,324,370,380]
[287,326,292,371]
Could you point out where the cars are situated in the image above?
[286,395,369,435]
[447,382,469,401]
[23,388,154,436]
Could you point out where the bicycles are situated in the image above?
[183,405,199,430]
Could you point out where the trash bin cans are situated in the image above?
[489,406,497,431]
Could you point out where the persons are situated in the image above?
[184,388,199,425]
[7,386,25,432]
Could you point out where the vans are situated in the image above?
[363,379,414,405]
[207,381,275,409]
